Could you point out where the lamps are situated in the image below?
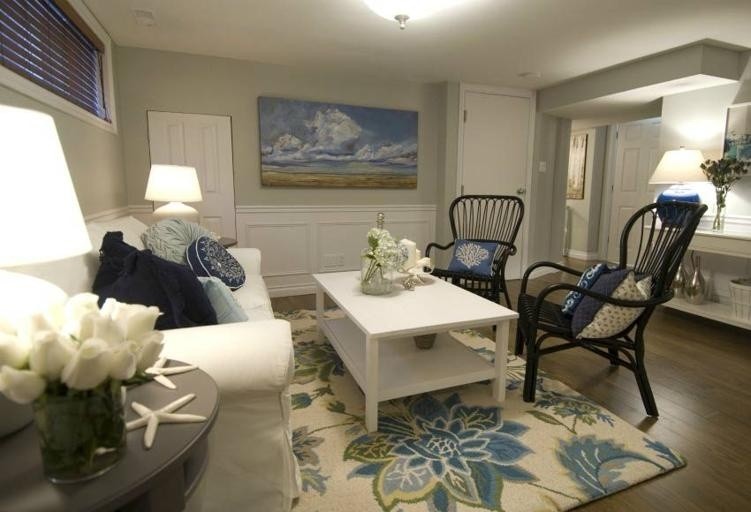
[143,163,204,225]
[648,146,712,230]
[0,102,94,283]
[360,0,466,30]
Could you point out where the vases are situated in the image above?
[712,188,731,232]
[672,255,689,297]
[359,262,394,295]
[683,249,706,305]
[36,384,131,488]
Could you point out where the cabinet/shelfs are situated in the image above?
[645,221,751,332]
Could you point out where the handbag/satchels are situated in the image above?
[92,230,219,328]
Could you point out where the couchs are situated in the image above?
[0,209,304,512]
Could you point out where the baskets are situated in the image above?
[729,278,751,325]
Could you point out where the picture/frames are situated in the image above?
[719,103,751,179]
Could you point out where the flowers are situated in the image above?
[0,287,172,405]
[701,157,751,187]
[361,224,410,272]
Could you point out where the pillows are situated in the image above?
[559,262,609,311]
[573,263,654,347]
[142,217,218,264]
[447,236,502,277]
[189,274,249,325]
[182,234,246,292]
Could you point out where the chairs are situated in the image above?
[419,193,527,329]
[514,201,709,420]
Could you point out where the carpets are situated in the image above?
[274,305,692,512]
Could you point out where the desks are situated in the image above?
[0,358,223,512]
